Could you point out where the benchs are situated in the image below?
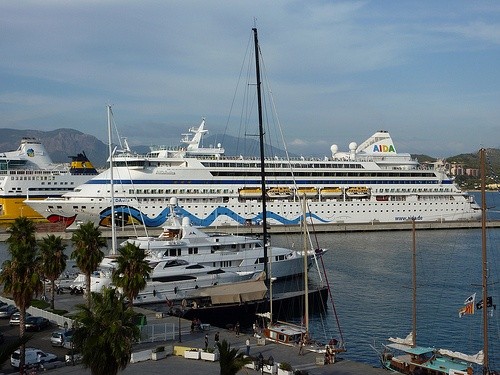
[195,323,210,330]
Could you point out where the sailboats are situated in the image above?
[368,147,500,375]
[250,13,348,357]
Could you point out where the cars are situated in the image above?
[0,304,18,319]
[9,312,20,327]
[24,316,50,332]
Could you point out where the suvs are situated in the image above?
[49,328,75,350]
[11,347,58,369]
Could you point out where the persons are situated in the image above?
[191,319,202,332]
[64,321,68,331]
[325,346,335,363]
[214,331,220,348]
[56,282,61,295]
[236,323,240,336]
[298,340,305,356]
[246,338,251,355]
[37,353,41,367]
[205,335,209,349]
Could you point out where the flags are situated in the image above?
[486,307,494,318]
[458,302,474,318]
[476,296,493,310]
[464,292,476,306]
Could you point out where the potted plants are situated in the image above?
[174,343,219,362]
[151,346,166,360]
[245,356,294,375]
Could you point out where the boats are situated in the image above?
[21,103,482,238]
[68,102,330,317]
[0,136,101,225]
